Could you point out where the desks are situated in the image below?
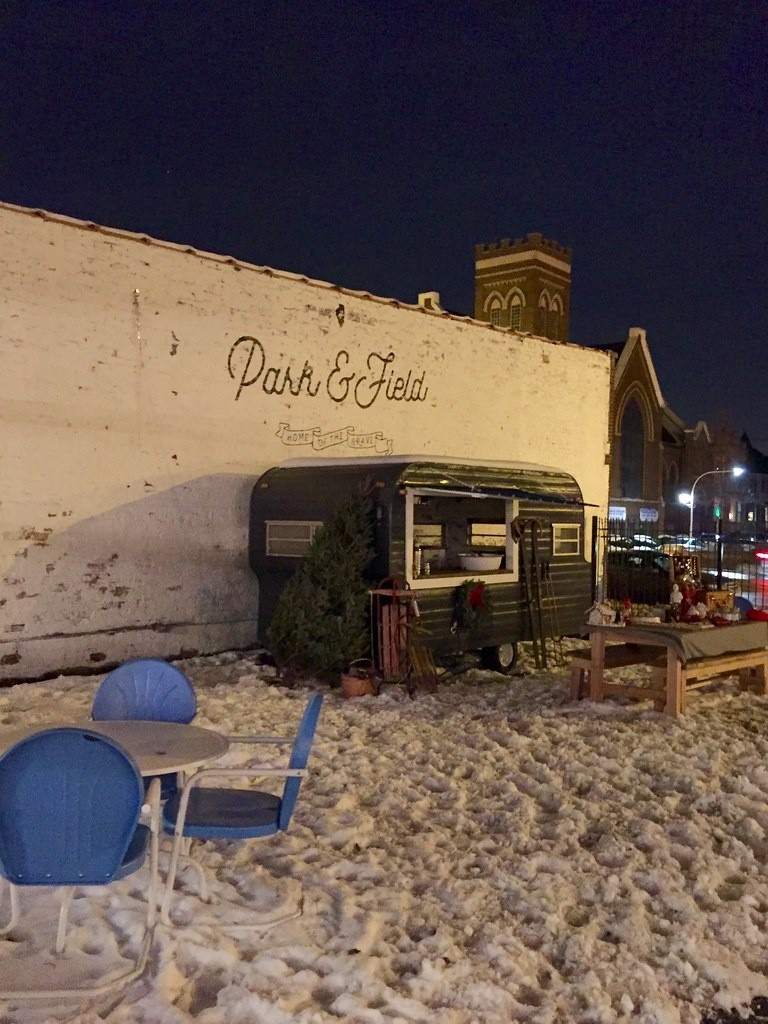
[578,618,768,713]
[2,718,229,955]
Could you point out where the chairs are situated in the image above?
[0,727,163,1001]
[77,658,204,872]
[159,691,326,931]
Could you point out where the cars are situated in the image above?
[606,531,749,598]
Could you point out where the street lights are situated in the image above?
[687,467,744,554]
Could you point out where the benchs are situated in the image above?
[644,647,768,718]
[562,642,668,702]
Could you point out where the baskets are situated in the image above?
[337,655,383,699]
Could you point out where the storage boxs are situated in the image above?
[669,552,735,618]
